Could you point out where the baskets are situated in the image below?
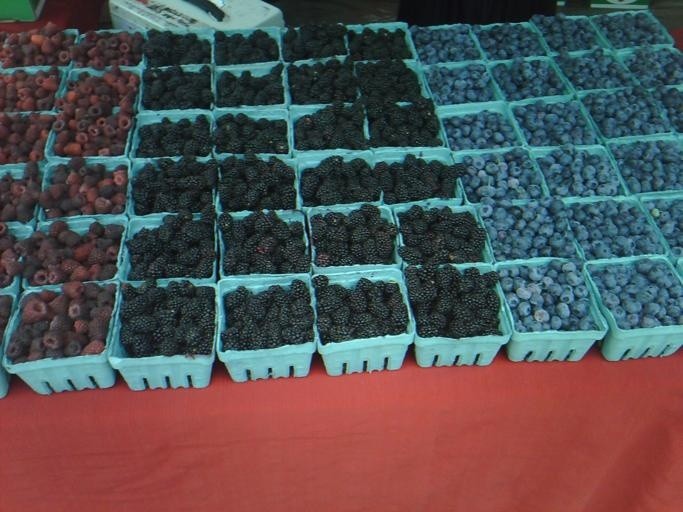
[0,8,683,401]
[0,8,683,397]
